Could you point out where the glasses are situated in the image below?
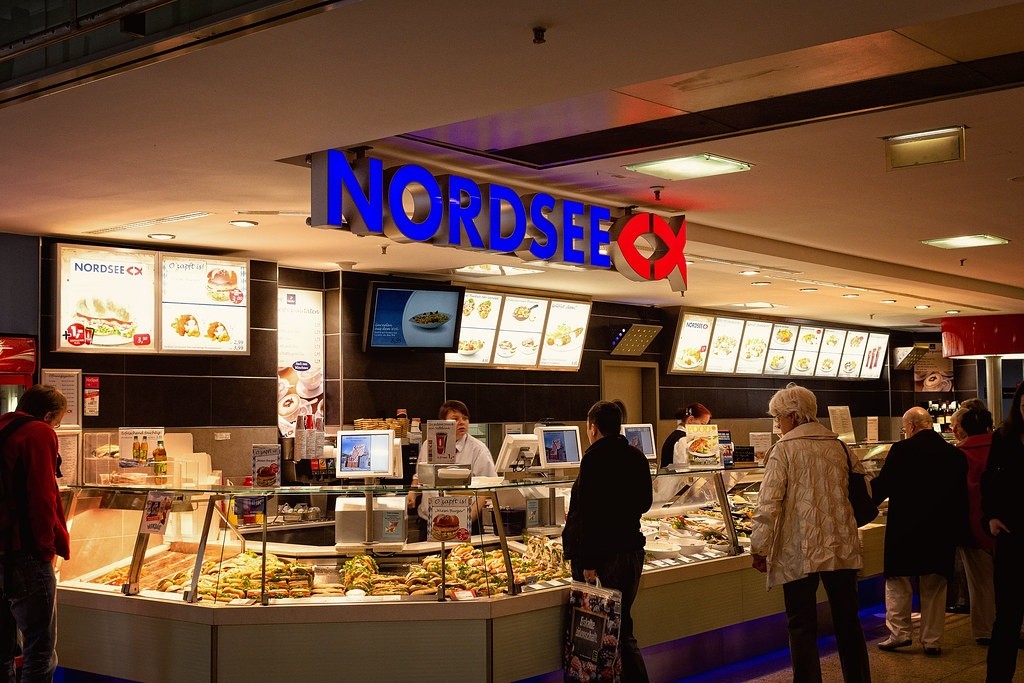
[774,415,783,422]
[901,423,911,432]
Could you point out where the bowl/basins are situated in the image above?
[519,341,537,354]
[669,537,705,555]
[457,338,482,358]
[497,345,517,357]
[644,542,681,561]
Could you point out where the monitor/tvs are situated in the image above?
[495,434,538,473]
[534,426,583,468]
[620,423,657,459]
[336,430,403,479]
[361,282,466,353]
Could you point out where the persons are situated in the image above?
[406,401,499,543]
[750,386,872,683]
[870,407,969,655]
[0,384,70,683]
[981,381,1024,683]
[949,398,992,648]
[561,401,653,683]
[658,402,712,509]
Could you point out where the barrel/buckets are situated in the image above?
[490,510,525,536]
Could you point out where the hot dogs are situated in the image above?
[311,582,347,597]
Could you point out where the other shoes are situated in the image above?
[923,644,940,653]
[878,637,913,648]
[946,604,971,613]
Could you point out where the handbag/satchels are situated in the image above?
[564,576,622,683]
[837,437,879,529]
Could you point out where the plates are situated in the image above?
[66,321,134,346]
[675,331,862,373]
[278,378,289,400]
[314,411,323,426]
[922,377,952,392]
[546,337,582,352]
[408,311,453,328]
[687,439,715,457]
[296,380,323,398]
[278,399,312,437]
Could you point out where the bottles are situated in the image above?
[926,399,961,432]
[132,436,140,460]
[140,435,148,460]
[154,441,167,484]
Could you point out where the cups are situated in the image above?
[923,373,947,392]
[279,437,294,460]
[296,362,323,390]
[317,399,323,416]
[294,415,324,460]
[278,394,308,422]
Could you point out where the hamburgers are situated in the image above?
[206,268,238,301]
[339,545,567,597]
[157,550,313,602]
[72,297,136,337]
[431,513,460,541]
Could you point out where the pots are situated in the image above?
[512,305,538,321]
